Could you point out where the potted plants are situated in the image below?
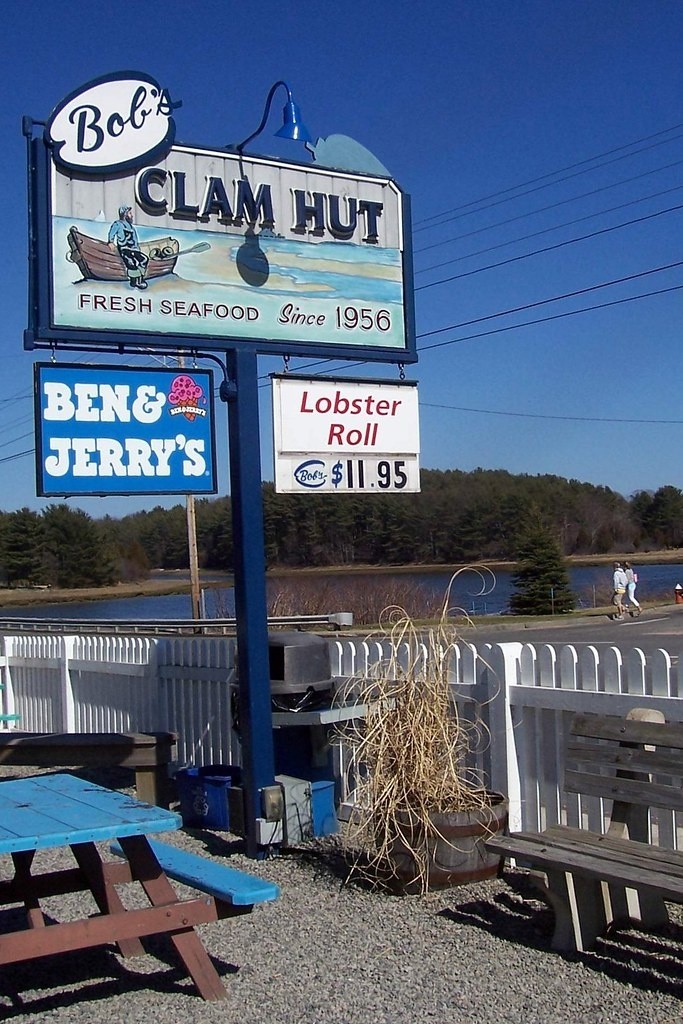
[330,564,509,897]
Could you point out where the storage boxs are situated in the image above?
[173,763,243,832]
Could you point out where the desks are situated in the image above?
[0,732,178,809]
[0,774,229,999]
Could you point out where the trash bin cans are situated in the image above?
[229,631,340,836]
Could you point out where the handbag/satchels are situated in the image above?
[634,574,637,582]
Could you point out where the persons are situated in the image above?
[621,561,644,616]
[610,562,629,620]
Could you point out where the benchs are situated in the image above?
[484,714,683,953]
[111,837,280,905]
